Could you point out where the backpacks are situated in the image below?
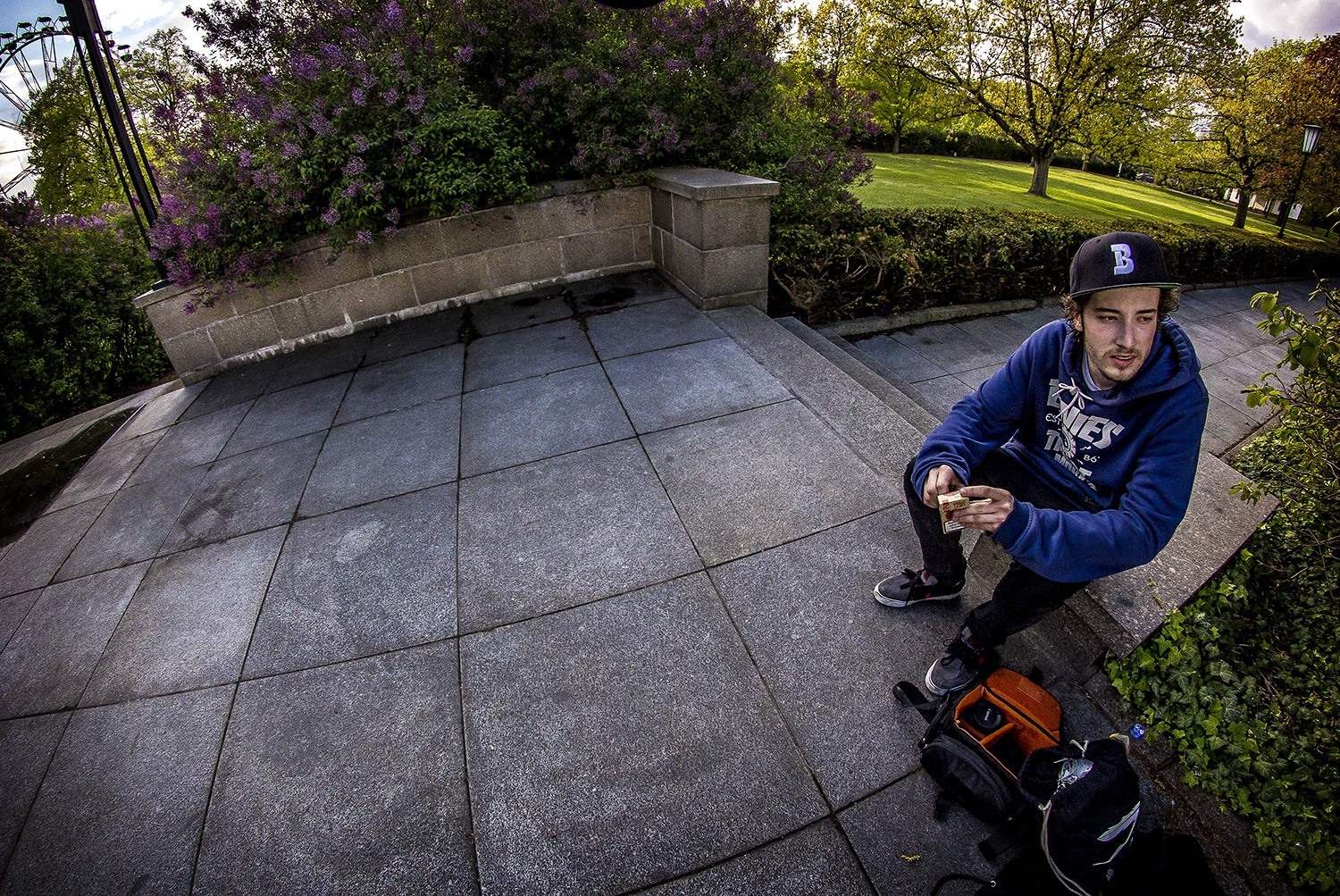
[892,666,1064,828]
[930,735,1141,896]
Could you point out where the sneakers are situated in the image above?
[923,606,1003,697]
[872,566,966,609]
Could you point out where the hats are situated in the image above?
[1066,230,1182,299]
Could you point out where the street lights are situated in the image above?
[1276,124,1324,240]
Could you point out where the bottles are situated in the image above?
[968,703,1004,736]
[1117,722,1145,753]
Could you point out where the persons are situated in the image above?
[872,232,1209,696]
